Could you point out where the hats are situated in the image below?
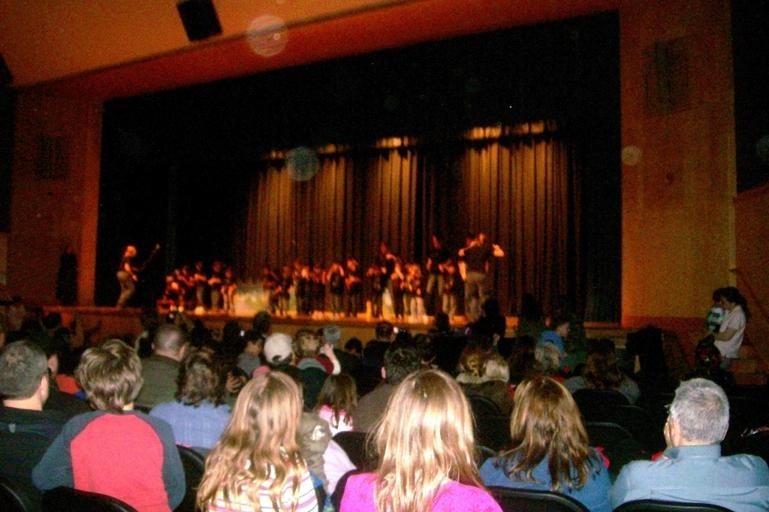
[537,329,565,353]
[263,332,293,365]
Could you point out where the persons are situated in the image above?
[115,244,139,308]
[157,231,505,322]
[0,285,769,511]
[56,244,78,306]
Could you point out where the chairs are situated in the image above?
[485,482,587,511]
[587,419,634,473]
[1,479,24,511]
[469,396,507,448]
[176,443,208,512]
[335,429,380,468]
[612,498,734,512]
[583,404,652,448]
[350,362,378,379]
[30,484,138,512]
[571,388,626,404]
